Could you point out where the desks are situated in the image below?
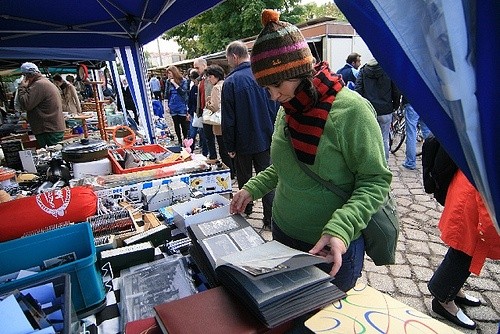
[82,223,315,334]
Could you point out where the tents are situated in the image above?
[0,48,150,137]
[0,0,228,145]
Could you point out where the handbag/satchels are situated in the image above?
[361,191,400,266]
[202,102,222,126]
[191,112,204,128]
[421,134,460,206]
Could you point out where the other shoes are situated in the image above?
[263,224,271,230]
[432,298,476,330]
[206,159,217,164]
[402,161,405,165]
[456,295,481,307]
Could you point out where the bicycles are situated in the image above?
[388,95,425,156]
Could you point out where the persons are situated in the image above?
[335,52,361,92]
[221,41,282,231]
[66,75,74,86]
[230,9,393,292]
[205,65,229,168]
[427,169,500,330]
[10,91,16,109]
[402,94,432,170]
[147,68,192,105]
[163,66,188,147]
[193,58,217,160]
[356,58,401,162]
[18,62,66,149]
[186,68,207,156]
[52,73,82,113]
[75,76,94,99]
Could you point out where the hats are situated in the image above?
[21,62,40,76]
[250,9,315,87]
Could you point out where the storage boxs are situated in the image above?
[108,143,184,175]
[0,272,81,334]
[141,180,170,212]
[1,139,26,172]
[0,221,108,319]
[0,132,30,145]
[85,209,141,248]
[188,159,233,198]
[19,150,38,174]
[158,206,175,224]
[166,180,191,202]
[72,157,113,178]
[172,193,232,237]
